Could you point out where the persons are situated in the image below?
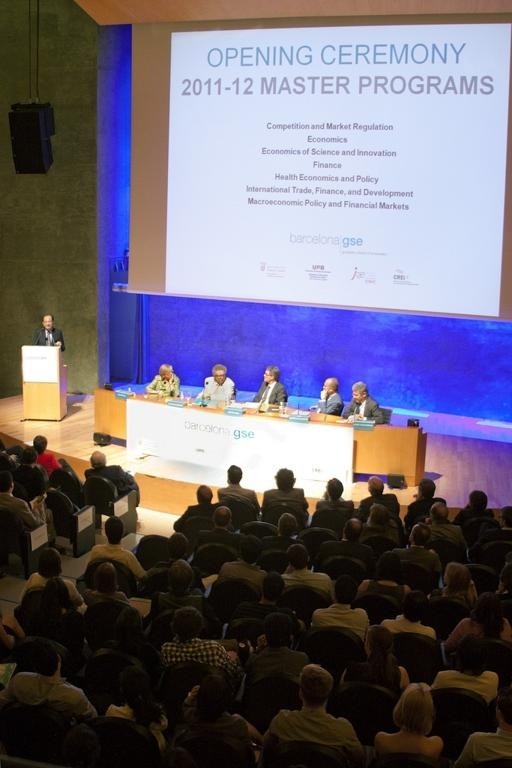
[313,377,343,415]
[341,381,384,425]
[196,364,236,406]
[32,313,65,353]
[145,364,180,400]
[0,435,512,766]
[253,365,287,406]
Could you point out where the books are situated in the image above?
[115,388,132,398]
[336,419,377,431]
[224,402,260,416]
[289,410,312,423]
[167,394,217,411]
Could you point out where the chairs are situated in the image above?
[1,448,511,768]
[377,406,393,423]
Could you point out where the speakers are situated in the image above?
[93,433,111,444]
[388,474,404,488]
[8,107,54,174]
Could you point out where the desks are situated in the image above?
[95,385,426,486]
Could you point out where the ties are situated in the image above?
[356,405,360,414]
[46,331,51,346]
[262,386,270,403]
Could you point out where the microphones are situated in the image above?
[200,382,208,407]
[353,402,362,422]
[45,338,53,345]
[324,394,330,422]
